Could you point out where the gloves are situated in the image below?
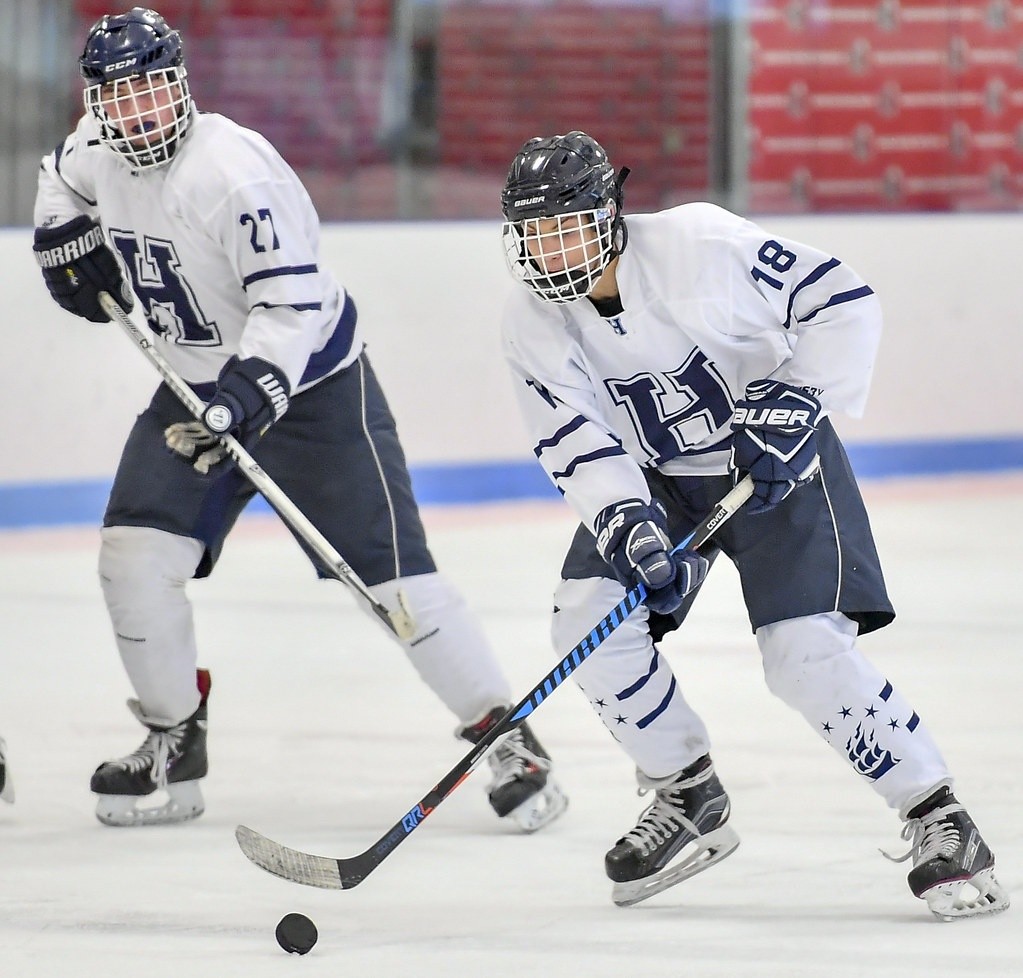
[592,498,709,614]
[163,353,292,482]
[32,215,134,323]
[728,378,820,516]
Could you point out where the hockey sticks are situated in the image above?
[233,468,756,893]
[97,287,419,644]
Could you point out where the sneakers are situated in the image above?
[90,668,211,827]
[605,752,740,908]
[877,777,1012,922]
[455,702,569,831]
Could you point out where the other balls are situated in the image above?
[274,912,320,955]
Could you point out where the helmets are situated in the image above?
[77,6,191,172]
[500,130,632,305]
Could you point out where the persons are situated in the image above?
[32,7,570,834]
[501,131,1011,924]
